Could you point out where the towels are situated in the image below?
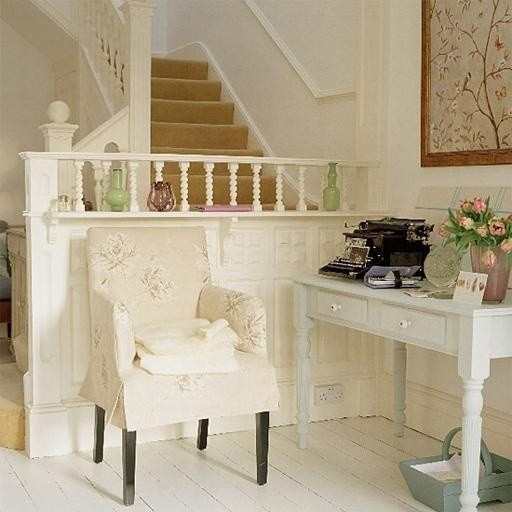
[129,317,240,379]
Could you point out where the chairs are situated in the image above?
[84,224,284,507]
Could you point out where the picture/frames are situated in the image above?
[420,0,512,167]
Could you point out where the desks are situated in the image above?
[285,270,512,512]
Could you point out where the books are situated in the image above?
[364,265,423,289]
[195,204,252,211]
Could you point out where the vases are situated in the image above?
[104,168,130,211]
[323,161,341,210]
[469,243,512,302]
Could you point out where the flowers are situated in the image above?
[438,192,512,270]
